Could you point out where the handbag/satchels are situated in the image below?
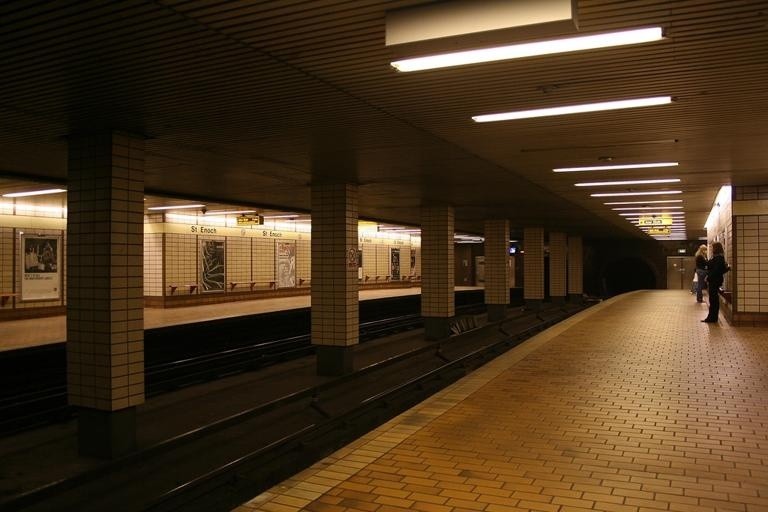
[723,262,730,274]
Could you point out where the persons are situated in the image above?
[690,267,707,295]
[24,247,57,273]
[694,244,709,302]
[700,241,729,323]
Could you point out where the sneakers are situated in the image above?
[700,316,718,323]
